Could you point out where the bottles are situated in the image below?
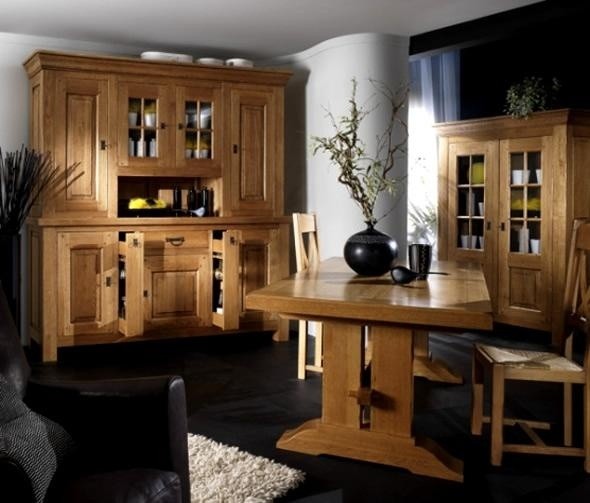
[129,138,156,157]
[173,184,209,217]
[466,193,475,217]
[519,229,529,252]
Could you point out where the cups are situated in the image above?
[478,202,483,216]
[479,236,483,249]
[461,235,478,249]
[409,243,432,280]
[512,169,530,184]
[531,239,539,253]
[194,150,208,159]
[144,112,156,127]
[536,169,541,184]
[186,149,193,159]
[129,111,137,126]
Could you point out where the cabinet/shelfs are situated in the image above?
[23,219,119,364]
[431,108,590,346]
[108,55,223,177]
[199,66,293,218]
[118,217,214,343]
[21,51,118,219]
[212,216,295,341]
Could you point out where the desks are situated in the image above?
[245,259,495,483]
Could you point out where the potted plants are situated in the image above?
[310,75,429,278]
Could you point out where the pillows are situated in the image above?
[0,373,76,503]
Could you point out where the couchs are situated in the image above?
[0,288,191,503]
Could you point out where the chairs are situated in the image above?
[293,213,373,380]
[470,223,590,473]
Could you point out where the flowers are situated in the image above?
[502,76,560,121]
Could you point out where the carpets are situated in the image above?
[186,432,307,503]
